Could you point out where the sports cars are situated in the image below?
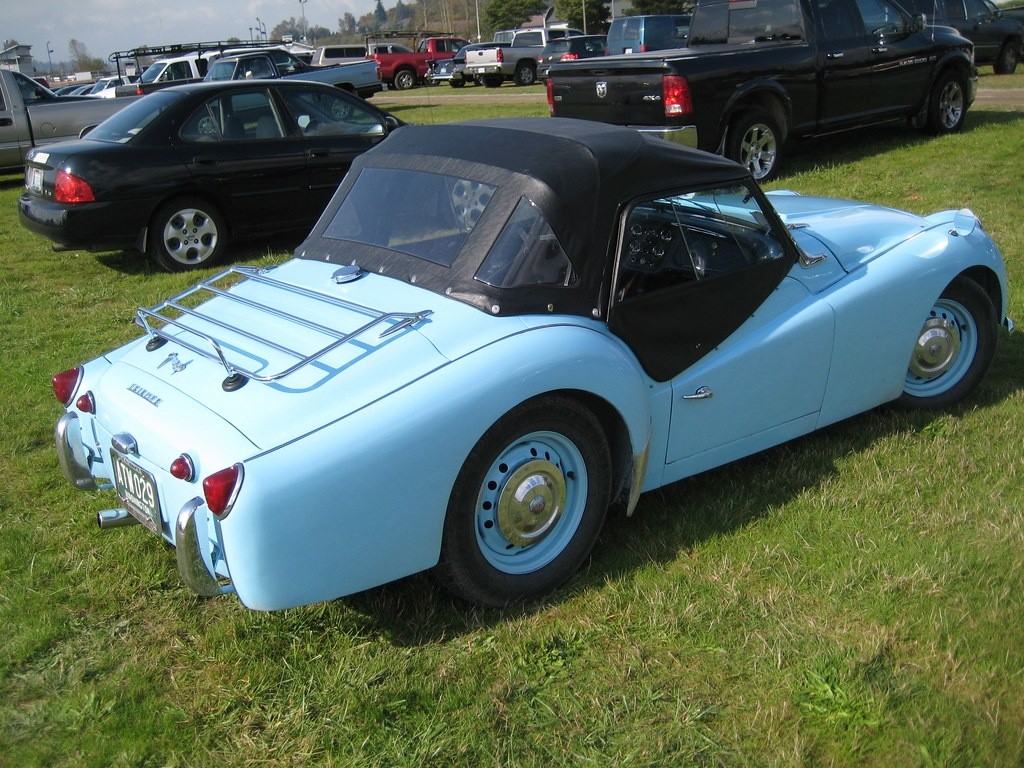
[52,117,1008,610]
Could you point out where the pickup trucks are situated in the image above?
[0,69,150,174]
[546,0,976,180]
[201,48,383,100]
[364,33,471,90]
[463,27,585,88]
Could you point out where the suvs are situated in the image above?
[537,36,606,87]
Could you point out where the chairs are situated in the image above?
[592,42,603,51]
[223,115,245,138]
[195,133,218,142]
[256,115,281,138]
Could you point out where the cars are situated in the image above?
[915,0,1024,74]
[47,41,292,135]
[429,41,512,89]
[18,79,411,274]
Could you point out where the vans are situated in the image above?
[311,43,413,66]
[605,15,703,56]
